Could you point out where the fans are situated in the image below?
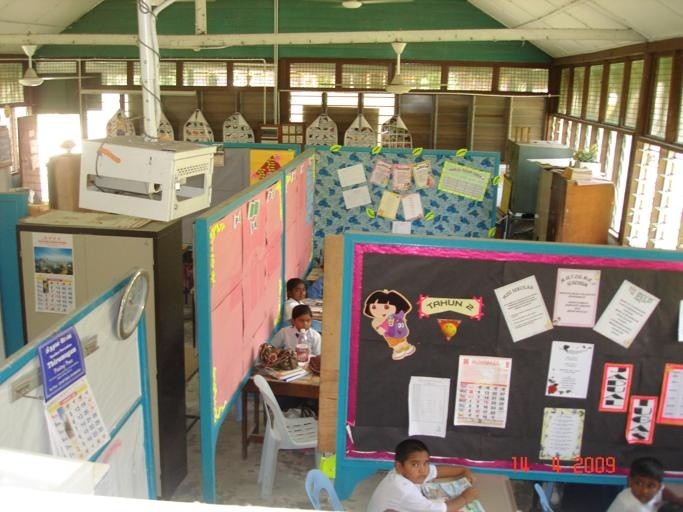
[2,43,97,88]
[319,43,459,98]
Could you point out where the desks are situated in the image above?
[242,360,322,458]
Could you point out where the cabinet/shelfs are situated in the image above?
[534,171,615,246]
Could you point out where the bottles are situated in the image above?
[295,328,310,369]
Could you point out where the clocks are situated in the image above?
[117,269,151,340]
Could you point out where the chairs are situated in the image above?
[304,468,347,511]
[534,484,557,512]
[255,375,318,492]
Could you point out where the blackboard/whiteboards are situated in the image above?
[336,232,683,485]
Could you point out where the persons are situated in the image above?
[281,279,309,325]
[605,456,683,512]
[364,439,481,512]
[272,304,322,418]
